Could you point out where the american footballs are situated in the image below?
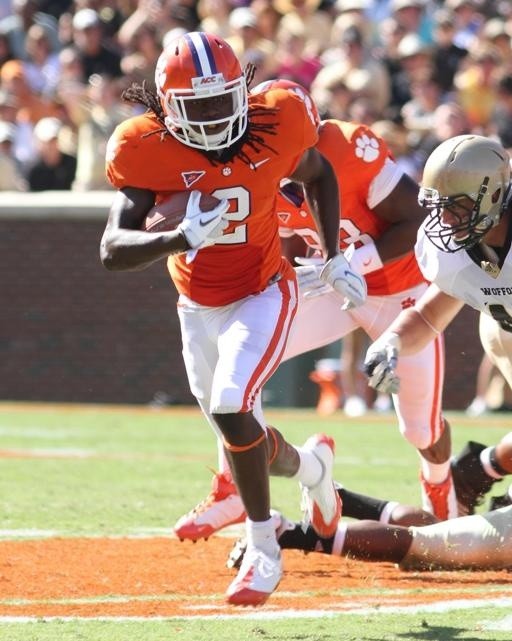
[140,196,218,232]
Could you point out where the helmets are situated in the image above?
[419,134,512,254]
[155,32,248,151]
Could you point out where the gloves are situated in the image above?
[293,257,335,300]
[319,254,368,312]
[361,333,401,395]
[179,190,229,251]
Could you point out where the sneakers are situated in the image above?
[451,441,502,518]
[304,434,342,538]
[173,473,246,544]
[420,468,458,521]
[227,508,290,574]
[226,546,284,606]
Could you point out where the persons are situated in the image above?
[171,77,460,542]
[223,479,512,579]
[240,0,369,114]
[0,0,152,191]
[363,132,512,514]
[99,30,367,609]
[370,0,512,183]
[152,1,240,56]
